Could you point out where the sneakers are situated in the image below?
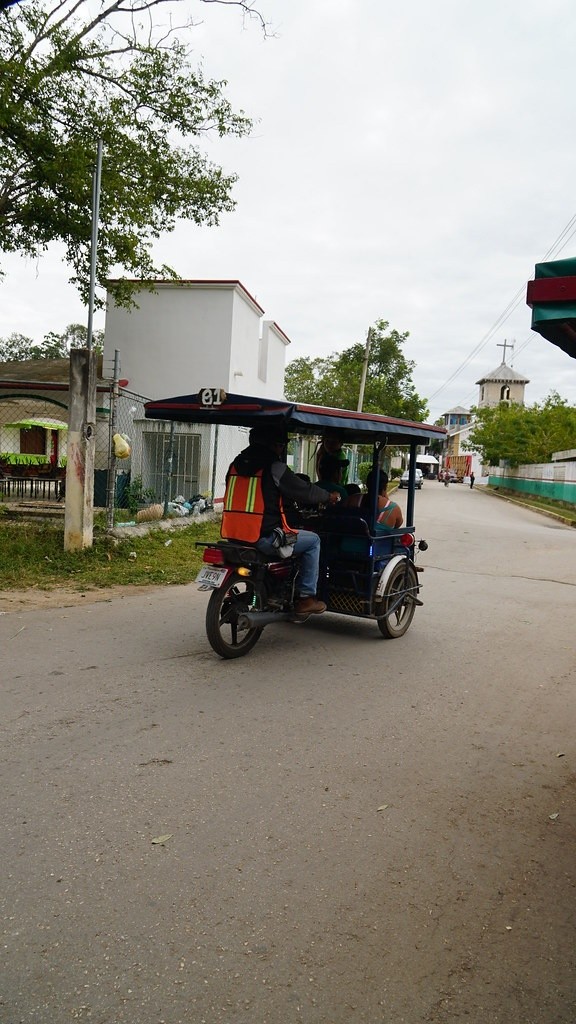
[294,596,327,616]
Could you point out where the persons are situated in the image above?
[291,431,403,571]
[225,426,340,615]
[443,470,450,487]
[470,472,475,489]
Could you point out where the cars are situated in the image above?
[439,466,460,482]
[400,467,426,490]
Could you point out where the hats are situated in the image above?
[249,427,290,448]
[318,453,349,470]
[342,484,367,496]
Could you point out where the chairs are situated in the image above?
[323,505,406,571]
[285,502,321,571]
